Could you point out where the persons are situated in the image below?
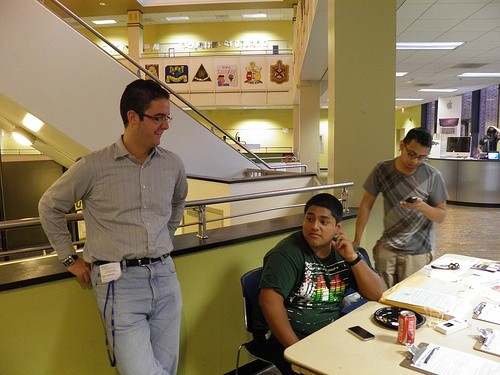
[38,79,189,375]
[257,194,383,350]
[480,126,500,152]
[352,127,447,288]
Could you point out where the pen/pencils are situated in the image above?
[425,348,436,364]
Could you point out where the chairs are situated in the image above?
[235,266,276,375]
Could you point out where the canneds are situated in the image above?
[398,310,416,345]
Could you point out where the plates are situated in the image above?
[374,306,427,329]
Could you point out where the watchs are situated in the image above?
[346,251,363,266]
[62,255,79,268]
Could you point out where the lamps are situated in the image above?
[12,127,36,147]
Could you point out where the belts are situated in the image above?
[91,248,173,270]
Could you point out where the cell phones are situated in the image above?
[348,326,375,340]
[406,197,417,203]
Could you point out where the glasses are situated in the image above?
[404,143,428,162]
[136,111,174,125]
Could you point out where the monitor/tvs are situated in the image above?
[446,136,472,156]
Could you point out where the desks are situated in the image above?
[283,253,500,375]
